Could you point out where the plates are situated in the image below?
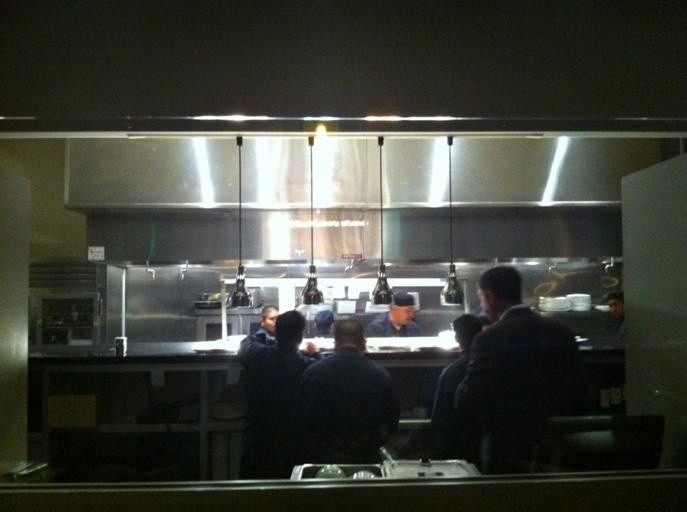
[195,300,221,308]
[594,305,611,313]
[537,293,592,312]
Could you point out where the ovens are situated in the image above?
[28,260,127,356]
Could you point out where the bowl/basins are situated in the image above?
[201,292,230,305]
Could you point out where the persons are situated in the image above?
[236,309,323,480]
[313,310,336,340]
[409,314,480,461]
[448,265,592,477]
[253,304,279,346]
[597,293,622,349]
[297,319,400,465]
[365,294,425,338]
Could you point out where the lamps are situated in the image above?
[368,136,398,309]
[300,135,325,307]
[438,135,466,308]
[225,136,252,308]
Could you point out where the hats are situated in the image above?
[315,310,333,330]
[602,289,623,300]
[392,293,414,306]
